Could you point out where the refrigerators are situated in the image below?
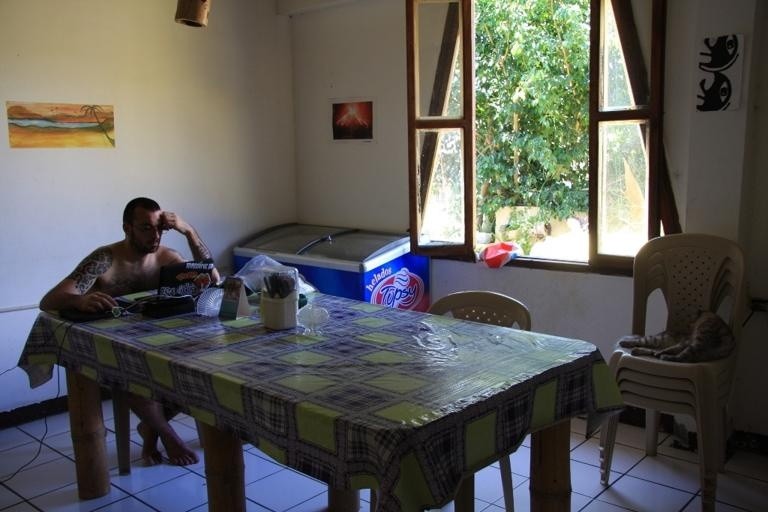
[232,221,429,314]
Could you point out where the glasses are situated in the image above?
[130,224,164,233]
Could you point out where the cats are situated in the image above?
[619,309,736,363]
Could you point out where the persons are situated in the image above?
[40,198,220,465]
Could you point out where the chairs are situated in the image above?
[596,233,747,510]
[370,290,530,511]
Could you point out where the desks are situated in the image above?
[36,287,597,511]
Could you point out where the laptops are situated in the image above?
[135,258,214,302]
